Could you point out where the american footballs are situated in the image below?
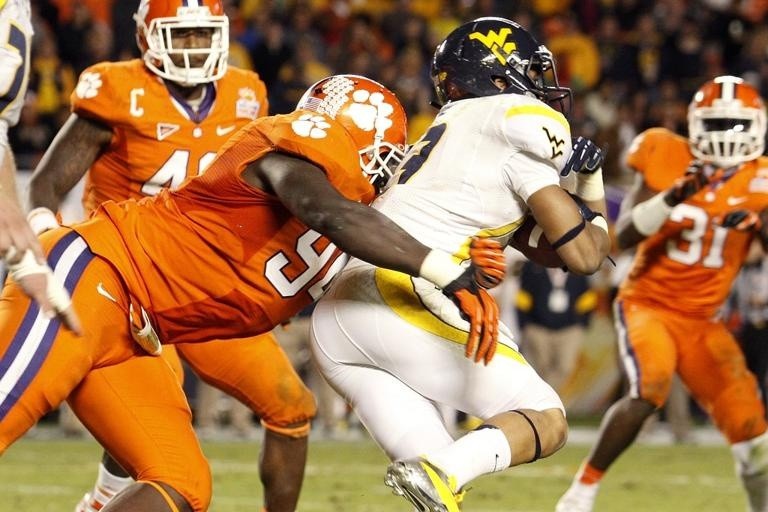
[515,190,587,269]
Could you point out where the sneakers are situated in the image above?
[554,458,600,512]
[384,454,474,512]
[75,492,104,512]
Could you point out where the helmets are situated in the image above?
[296,74,407,198]
[428,17,574,122]
[686,74,768,169]
[135,0,231,88]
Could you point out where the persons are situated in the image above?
[8,1,141,174]
[23,0,322,512]
[486,1,767,442]
[223,1,487,145]
[307,15,613,511]
[553,72,768,512]
[285,246,527,442]
[0,73,507,512]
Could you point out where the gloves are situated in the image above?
[442,267,500,366]
[717,208,768,240]
[560,135,604,178]
[468,237,506,290]
[662,158,724,208]
[564,189,604,223]
